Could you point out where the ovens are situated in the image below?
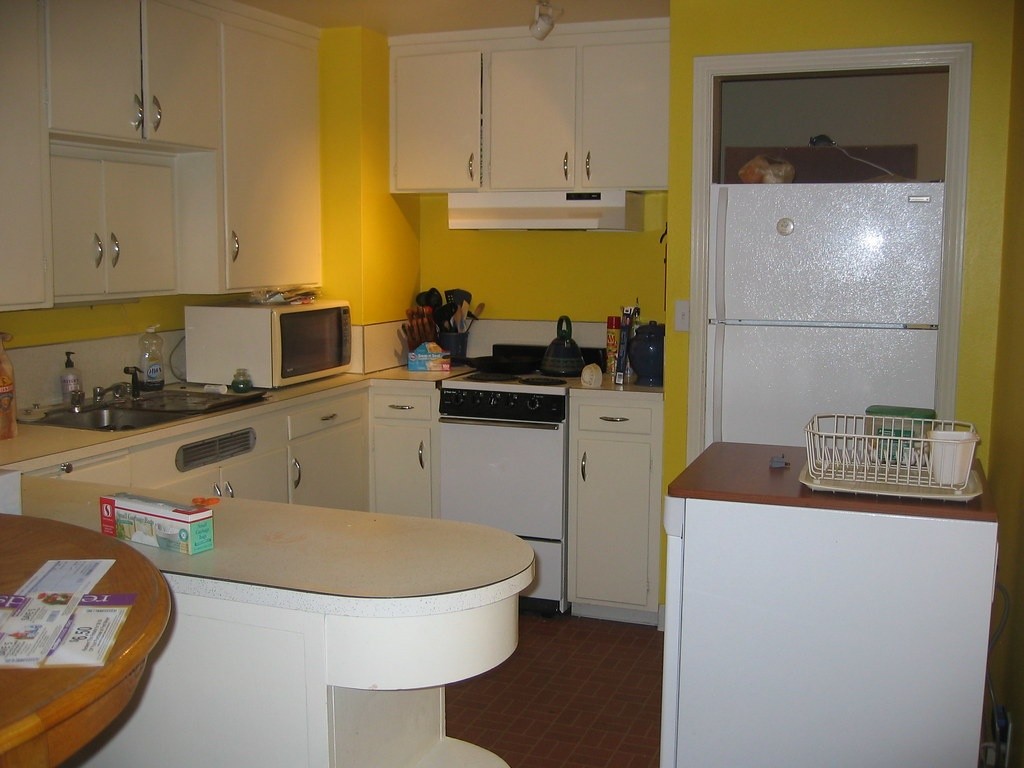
[439,418,571,619]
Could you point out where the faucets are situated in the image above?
[93,382,132,405]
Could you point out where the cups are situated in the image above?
[438,331,469,367]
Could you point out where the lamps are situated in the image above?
[528,0,564,41]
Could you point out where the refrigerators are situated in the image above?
[659,442,999,768]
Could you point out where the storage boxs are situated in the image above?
[99,492,215,555]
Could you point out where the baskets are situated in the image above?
[804,413,981,490]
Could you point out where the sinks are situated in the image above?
[45,409,176,430]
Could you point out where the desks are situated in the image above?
[0,513,173,768]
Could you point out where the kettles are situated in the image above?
[540,315,585,377]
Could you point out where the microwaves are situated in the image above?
[184,298,352,390]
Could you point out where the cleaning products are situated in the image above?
[138,323,167,391]
[60,350,83,405]
[0,330,19,440]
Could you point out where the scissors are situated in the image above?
[191,498,220,507]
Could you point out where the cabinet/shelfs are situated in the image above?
[488,26,667,191]
[567,388,665,626]
[286,387,370,512]
[49,143,177,303]
[369,379,435,518]
[46,0,220,153]
[152,447,287,504]
[174,0,323,295]
[389,40,489,194]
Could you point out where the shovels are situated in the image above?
[454,305,461,333]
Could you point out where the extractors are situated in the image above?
[447,189,646,233]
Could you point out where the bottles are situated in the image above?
[232,369,251,393]
[138,323,164,392]
[0,331,18,441]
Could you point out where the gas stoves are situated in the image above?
[439,344,607,424]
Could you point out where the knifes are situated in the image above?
[402,306,433,343]
